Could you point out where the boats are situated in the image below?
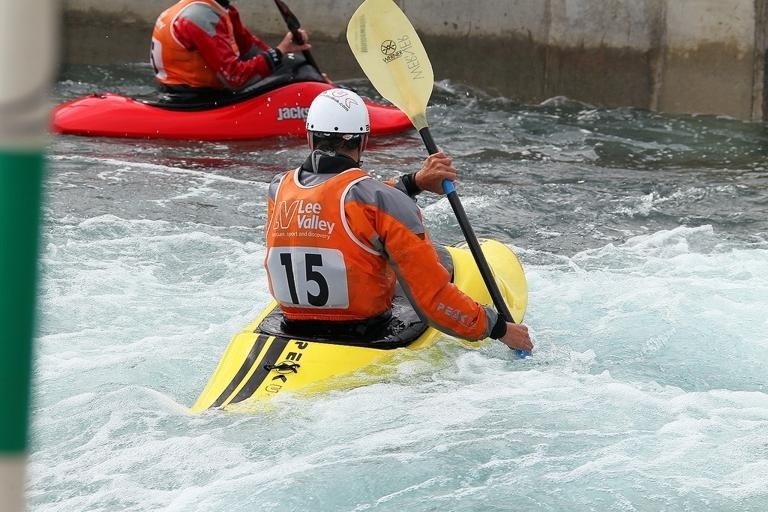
[189,237,528,414]
[50,53,413,140]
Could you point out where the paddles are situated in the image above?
[347,0,530,359]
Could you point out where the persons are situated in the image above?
[262,88,534,352]
[149,0,311,91]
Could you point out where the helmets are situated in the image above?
[305,88,370,134]
